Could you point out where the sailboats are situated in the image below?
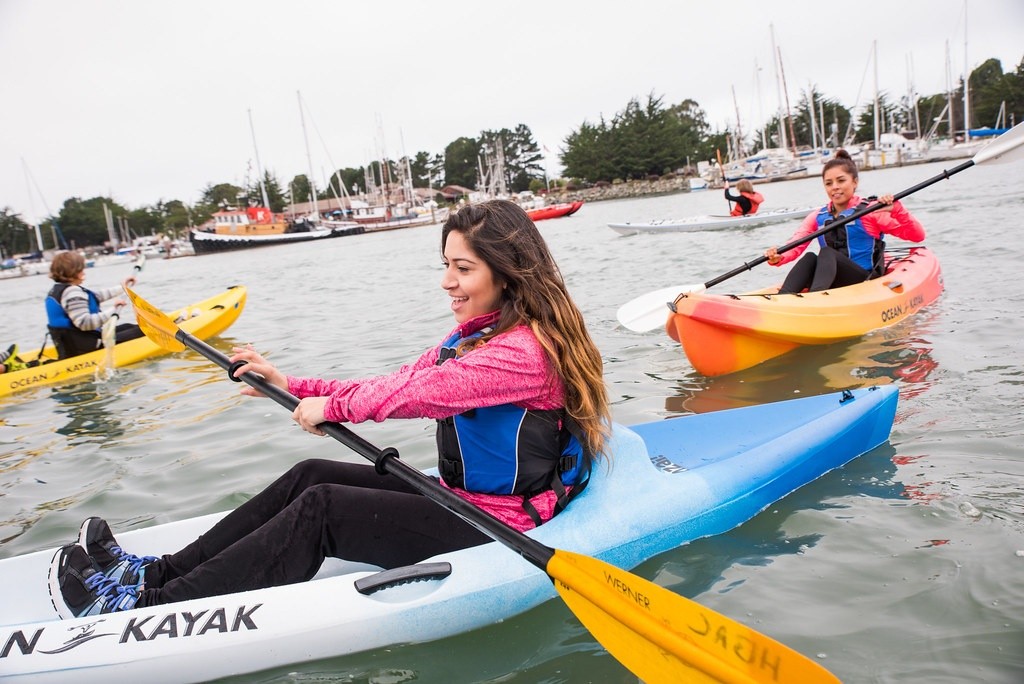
[694,27,1024,189]
[0,93,587,281]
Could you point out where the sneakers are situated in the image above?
[5,359,41,373]
[0,344,18,364]
[47,516,160,620]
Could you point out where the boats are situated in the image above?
[1,384,898,684]
[603,207,819,235]
[0,286,250,395]
[665,243,948,378]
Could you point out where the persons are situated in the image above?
[0,342,17,373]
[723,178,764,218]
[47,201,617,620]
[44,250,146,357]
[510,197,520,207]
[764,145,925,294]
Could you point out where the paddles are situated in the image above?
[117,286,847,684]
[715,149,733,212]
[101,254,147,351]
[615,117,1024,335]
